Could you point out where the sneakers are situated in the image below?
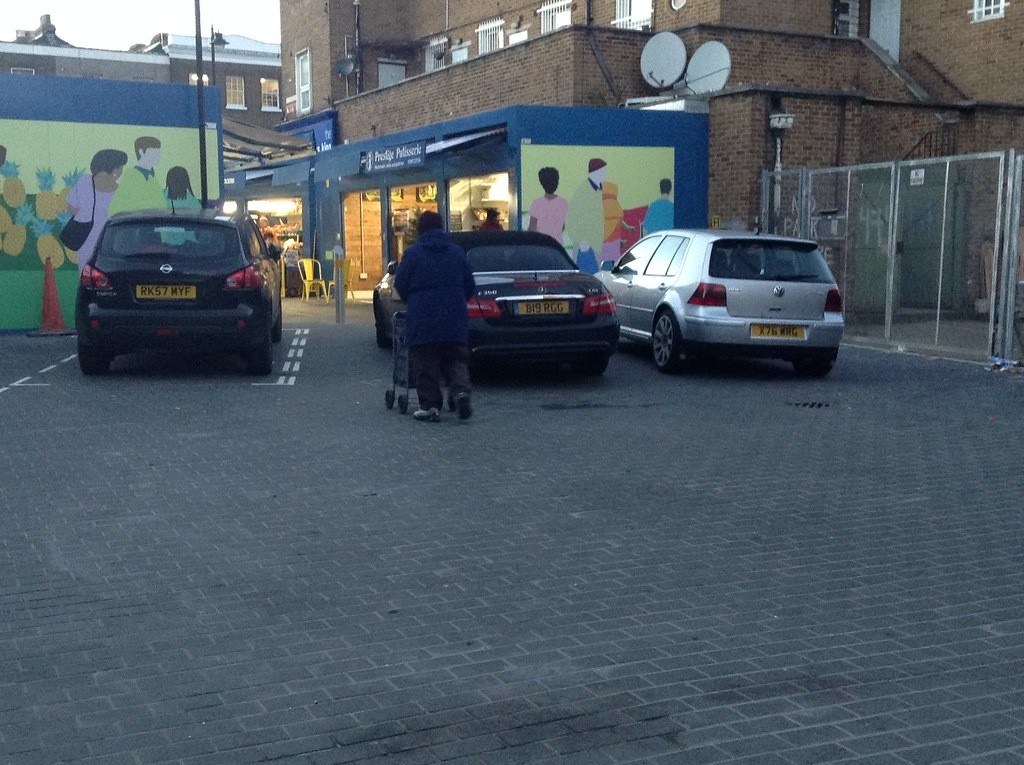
[413,406,441,424]
[450,390,471,421]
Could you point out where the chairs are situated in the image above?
[297,258,328,302]
[326,259,356,303]
[122,228,223,255]
[723,255,799,280]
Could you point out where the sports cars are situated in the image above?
[369,231,620,376]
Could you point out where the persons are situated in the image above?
[261,233,283,264]
[479,209,504,231]
[395,210,476,423]
[256,216,278,245]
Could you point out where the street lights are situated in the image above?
[209,25,228,91]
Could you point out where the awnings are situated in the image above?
[222,116,317,171]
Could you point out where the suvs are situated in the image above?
[584,228,844,379]
[74,207,288,377]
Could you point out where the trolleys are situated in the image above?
[381,311,463,414]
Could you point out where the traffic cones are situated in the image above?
[27,255,81,338]
